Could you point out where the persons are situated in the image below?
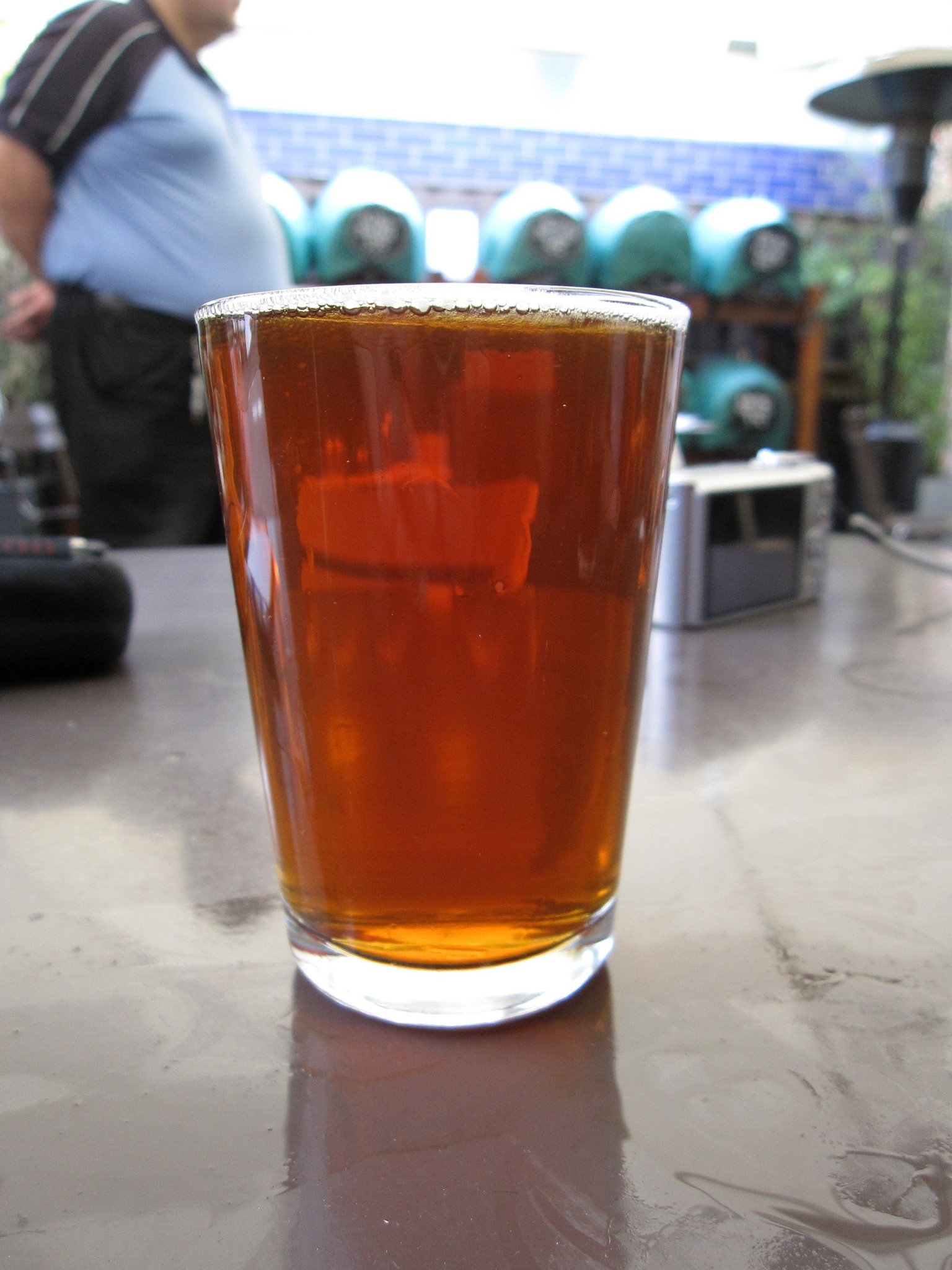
[0,0,291,549]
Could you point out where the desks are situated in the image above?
[1,526,949,1267]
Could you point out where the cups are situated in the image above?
[193,281,690,1033]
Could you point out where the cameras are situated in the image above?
[651,454,838,628]
